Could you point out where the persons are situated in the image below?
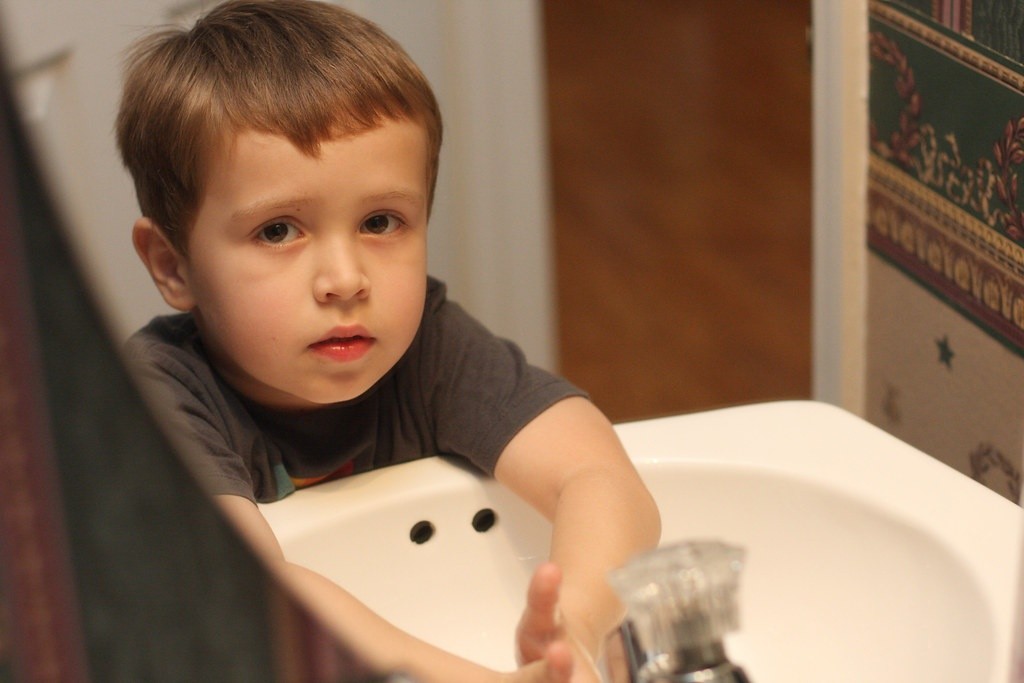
[114,0,660,682]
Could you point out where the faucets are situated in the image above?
[591,537,750,683]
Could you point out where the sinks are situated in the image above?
[246,401,1024,683]
